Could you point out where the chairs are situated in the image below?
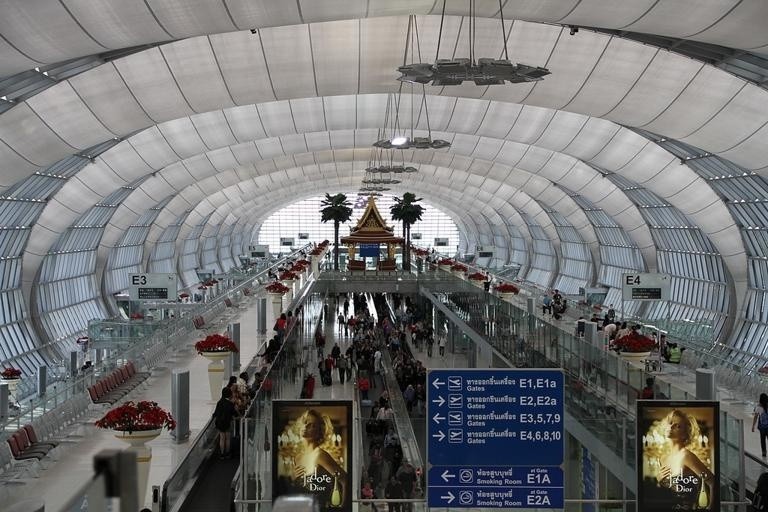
[713,365,767,415]
[84,327,199,411]
[192,273,270,335]
[0,394,107,492]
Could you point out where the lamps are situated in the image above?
[357,1,552,198]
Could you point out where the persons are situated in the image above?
[81,360,94,375]
[656,408,715,510]
[751,392,768,457]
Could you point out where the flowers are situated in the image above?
[415,249,485,280]
[266,259,310,293]
[611,331,657,353]
[0,366,22,379]
[193,331,239,356]
[93,398,178,435]
[309,240,329,255]
[494,284,519,293]
